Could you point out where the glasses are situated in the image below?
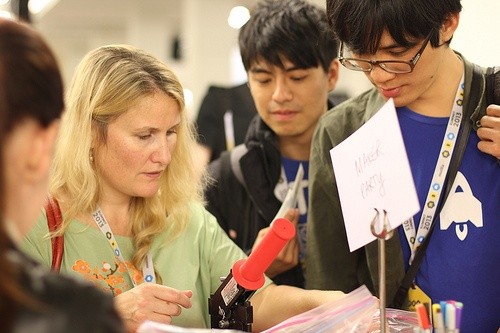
[338,27,437,74]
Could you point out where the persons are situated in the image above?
[203,0,341,287]
[304,0,500,333]
[0,20,123,333]
[20,44,344,333]
